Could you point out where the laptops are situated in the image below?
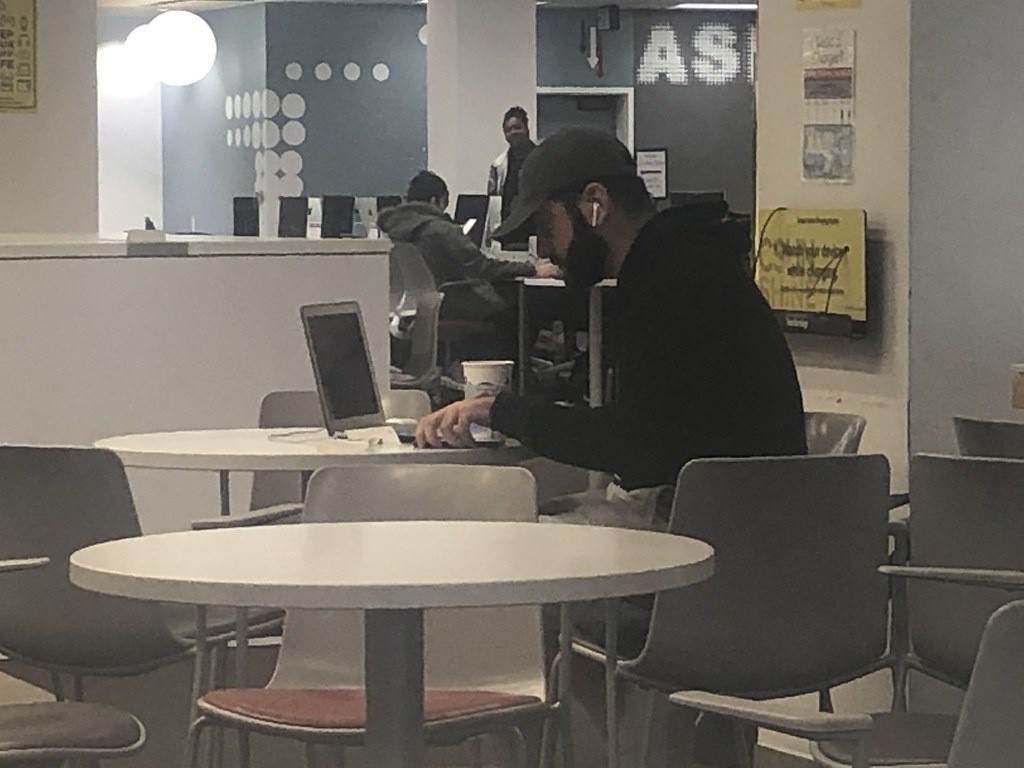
[300,301,418,442]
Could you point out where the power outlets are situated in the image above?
[1010,363,1024,410]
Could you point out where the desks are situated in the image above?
[93,426,541,533]
[507,274,619,409]
[68,514,717,768]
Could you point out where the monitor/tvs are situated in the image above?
[376,195,404,238]
[278,195,308,238]
[233,196,259,236]
[452,194,492,252]
[321,195,356,238]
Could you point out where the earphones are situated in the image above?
[591,200,600,227]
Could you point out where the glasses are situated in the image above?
[442,200,448,207]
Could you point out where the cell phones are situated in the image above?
[413,436,506,449]
[462,218,478,236]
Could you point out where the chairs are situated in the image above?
[0,389,1024,768]
[387,237,497,387]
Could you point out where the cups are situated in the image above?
[461,361,515,442]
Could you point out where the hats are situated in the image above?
[488,128,637,244]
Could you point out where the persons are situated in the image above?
[375,170,564,392]
[413,126,808,768]
[486,106,539,245]
